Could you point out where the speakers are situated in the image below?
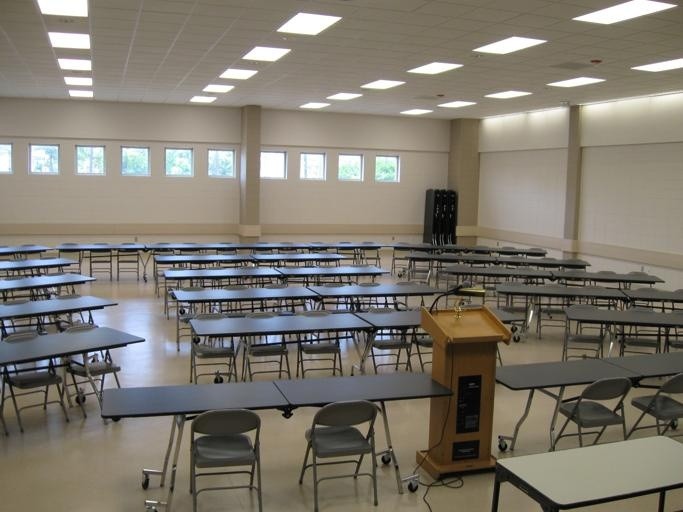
[423,189,457,243]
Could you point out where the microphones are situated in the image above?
[429,280,472,313]
